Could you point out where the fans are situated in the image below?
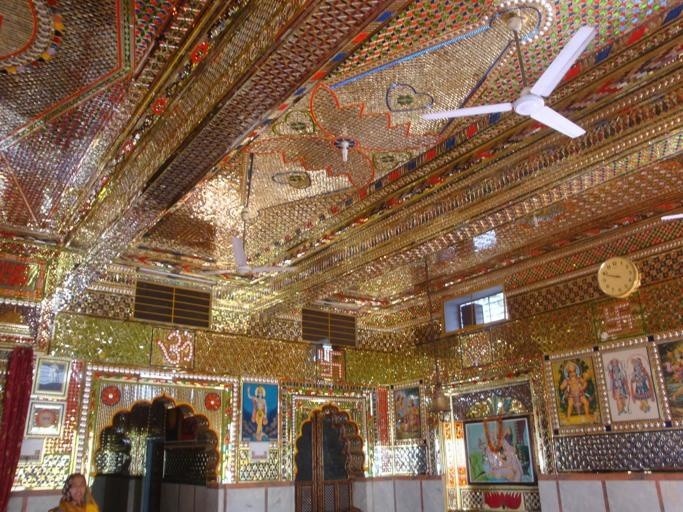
[419,13,597,140]
[199,208,297,278]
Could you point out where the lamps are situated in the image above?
[423,255,451,424]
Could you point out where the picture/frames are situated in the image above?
[462,414,537,486]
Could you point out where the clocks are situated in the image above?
[596,255,640,299]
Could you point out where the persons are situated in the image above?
[57,474,100,512]
[504,434,522,482]
[246,385,267,442]
